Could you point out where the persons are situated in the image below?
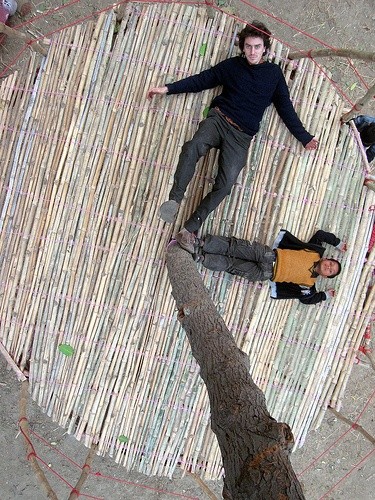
[146,19,321,253]
[187,229,348,305]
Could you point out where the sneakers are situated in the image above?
[175,228,195,254]
[194,237,204,248]
[159,200,178,222]
[192,252,204,263]
